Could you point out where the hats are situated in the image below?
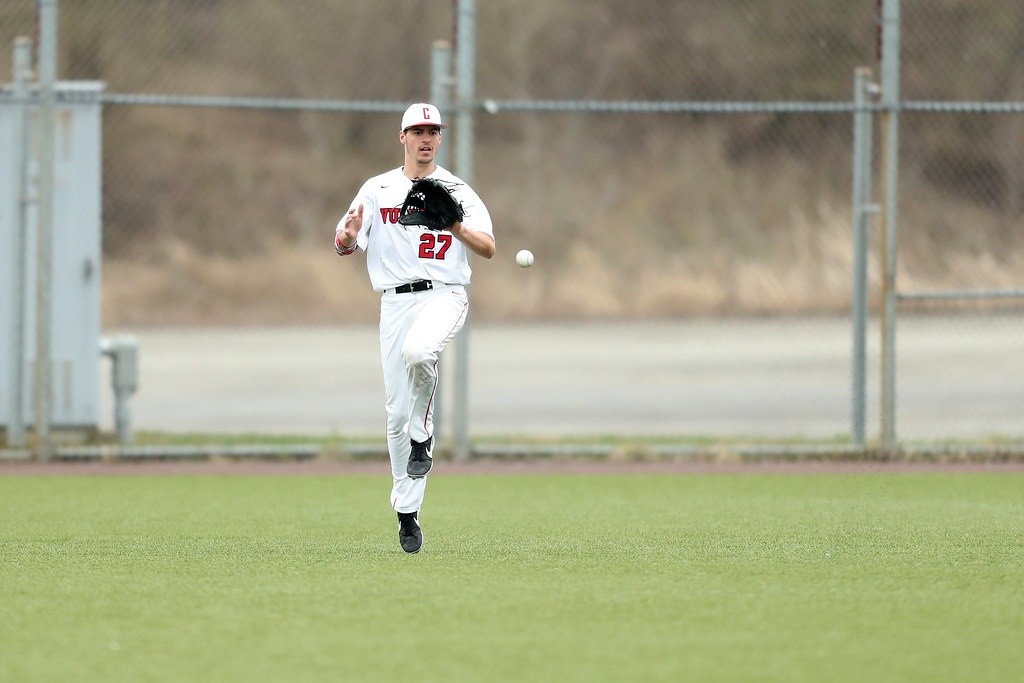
[401,103,448,132]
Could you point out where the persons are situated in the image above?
[334,103,496,554]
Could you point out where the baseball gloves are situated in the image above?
[396,176,466,230]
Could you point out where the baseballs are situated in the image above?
[515,249,535,268]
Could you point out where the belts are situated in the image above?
[384,280,461,294]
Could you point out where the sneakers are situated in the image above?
[396,506,424,554]
[407,435,435,480]
